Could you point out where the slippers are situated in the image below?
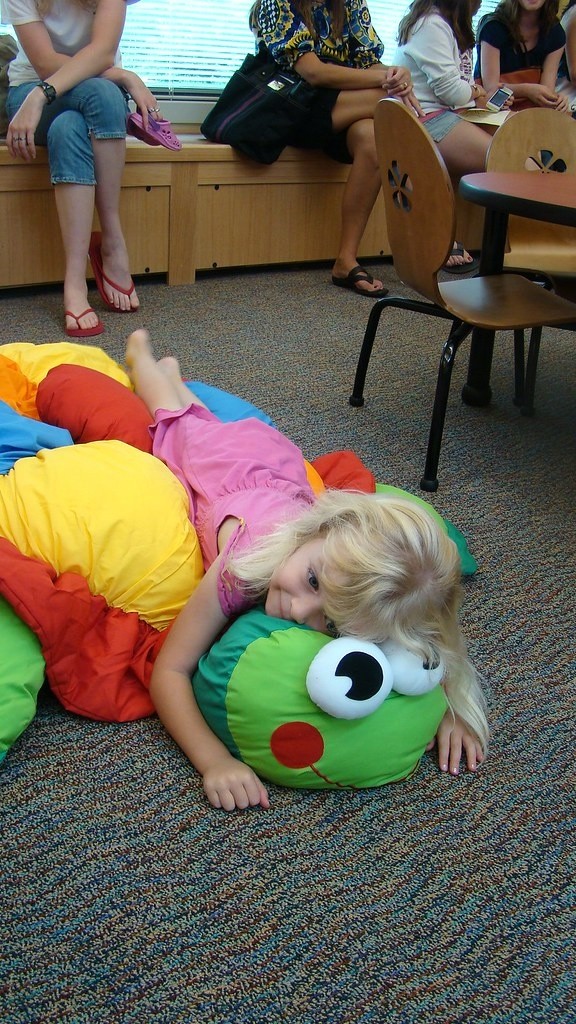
[127,112,160,147]
[131,112,182,151]
[90,231,139,312]
[331,266,389,299]
[65,308,105,337]
[442,241,478,273]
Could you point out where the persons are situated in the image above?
[394,0,576,177]
[127,327,490,811]
[248,0,479,297]
[2,0,163,337]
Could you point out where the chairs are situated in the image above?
[485,106,576,416]
[349,98,575,489]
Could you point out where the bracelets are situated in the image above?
[472,85,477,90]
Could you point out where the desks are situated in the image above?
[456,174,575,411]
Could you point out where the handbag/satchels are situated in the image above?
[201,53,321,166]
[475,68,557,137]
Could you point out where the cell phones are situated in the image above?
[485,86,514,112]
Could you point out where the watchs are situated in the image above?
[37,81,56,105]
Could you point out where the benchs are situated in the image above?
[0,132,496,291]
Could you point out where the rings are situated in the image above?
[404,83,407,87]
[19,137,26,141]
[13,136,19,143]
[148,108,155,112]
[155,108,160,112]
